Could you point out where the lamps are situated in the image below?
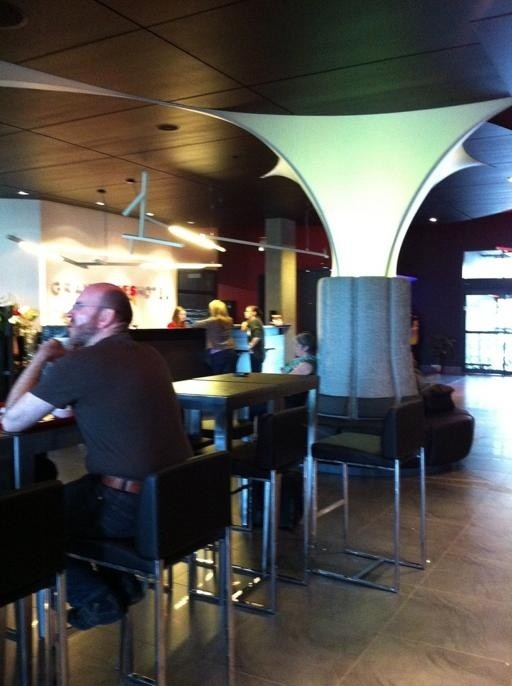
[6,209,229,273]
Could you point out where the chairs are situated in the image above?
[52,449,244,686]
[307,397,429,596]
[173,405,310,616]
[306,380,475,475]
[1,476,74,685]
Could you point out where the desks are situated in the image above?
[168,370,323,587]
[1,401,89,686]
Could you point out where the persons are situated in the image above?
[240,305,266,372]
[410,315,463,410]
[191,299,236,374]
[1,282,191,629]
[239,331,316,443]
[14,301,20,314]
[168,305,189,328]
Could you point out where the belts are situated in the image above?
[102,475,143,496]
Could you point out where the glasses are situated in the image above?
[73,303,121,316]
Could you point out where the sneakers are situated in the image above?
[68,592,122,630]
